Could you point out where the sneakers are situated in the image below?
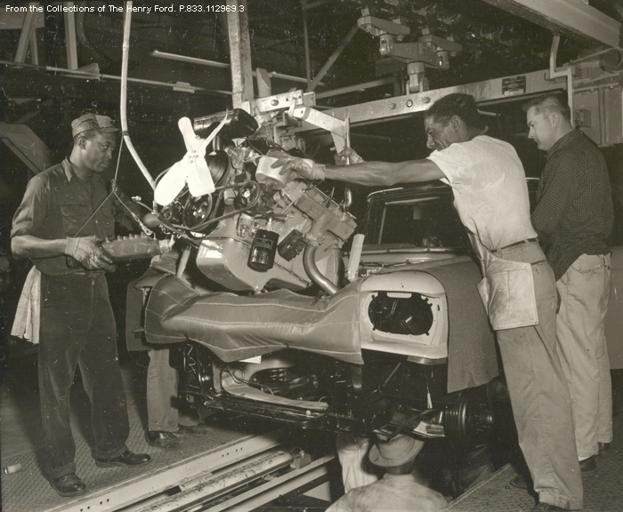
[510,440,609,512]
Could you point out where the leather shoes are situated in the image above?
[93,450,150,469]
[150,431,187,449]
[49,471,84,496]
[180,424,213,436]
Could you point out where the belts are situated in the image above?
[56,271,103,279]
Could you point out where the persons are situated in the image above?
[270,90,585,511]
[141,348,215,452]
[520,92,616,473]
[8,112,165,497]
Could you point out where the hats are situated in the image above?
[70,114,120,139]
